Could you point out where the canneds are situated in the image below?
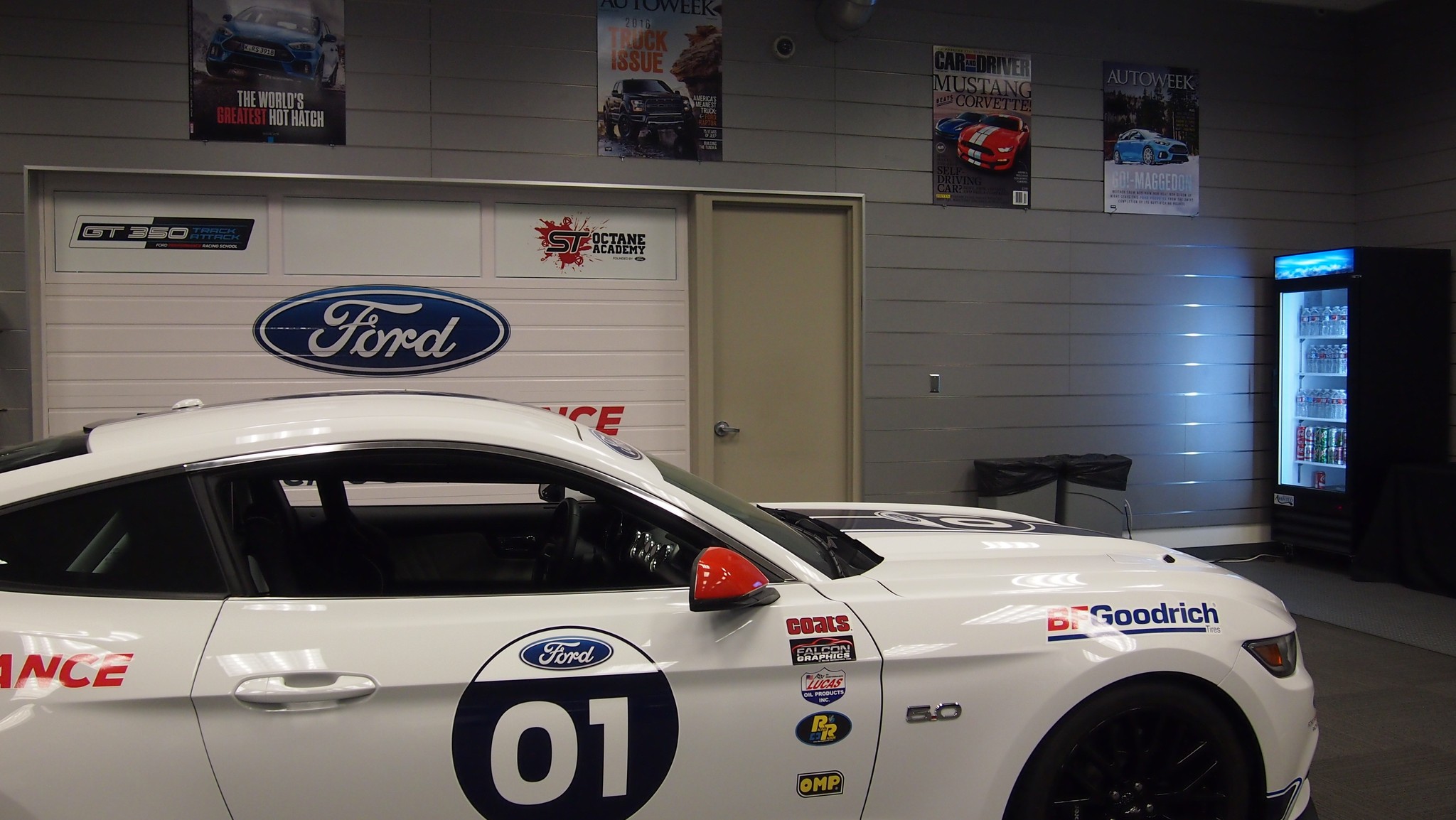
[1297,426,1346,465]
[1315,471,1326,489]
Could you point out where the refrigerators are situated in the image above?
[1268,246,1451,557]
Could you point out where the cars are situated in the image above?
[2,391,1321,820]
[205,6,340,88]
[1113,129,1189,165]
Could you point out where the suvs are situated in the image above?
[603,79,692,139]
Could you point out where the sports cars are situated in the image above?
[957,114,1030,171]
[935,111,987,142]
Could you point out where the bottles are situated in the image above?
[1300,306,1348,336]
[1307,343,1348,374]
[1296,387,1347,420]
